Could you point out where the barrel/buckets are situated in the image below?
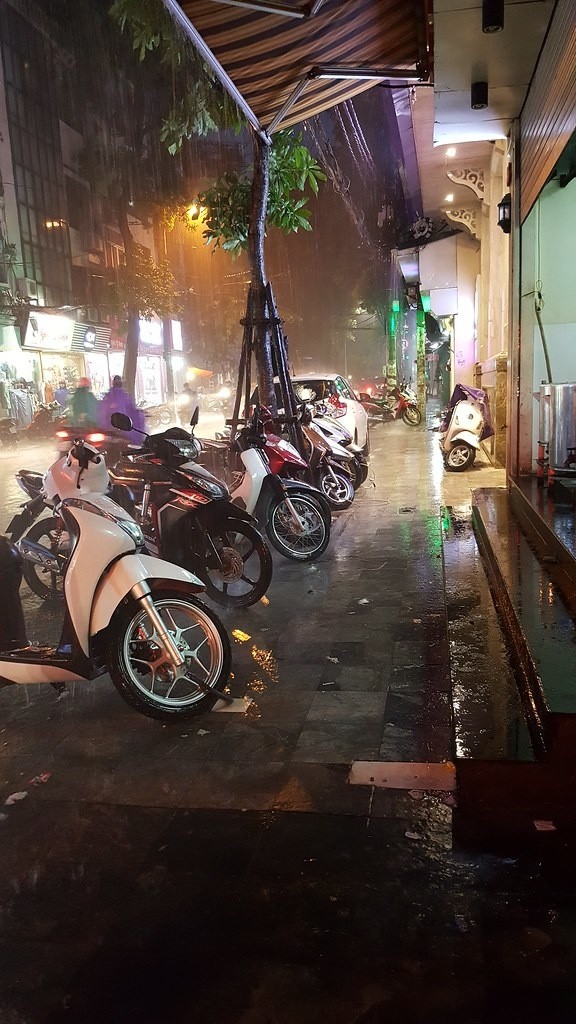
[533,383,576,467]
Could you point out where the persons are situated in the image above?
[45,385,53,402]
[183,383,196,396]
[97,376,145,445]
[73,379,97,417]
[221,379,234,419]
[55,380,70,411]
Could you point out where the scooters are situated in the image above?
[0,432,233,725]
[437,385,487,473]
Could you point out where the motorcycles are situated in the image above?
[351,376,423,427]
[126,395,368,563]
[0,379,273,612]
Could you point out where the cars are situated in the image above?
[244,372,372,462]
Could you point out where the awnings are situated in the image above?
[164,0,432,145]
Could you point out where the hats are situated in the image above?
[114,376,121,380]
[80,377,90,386]
